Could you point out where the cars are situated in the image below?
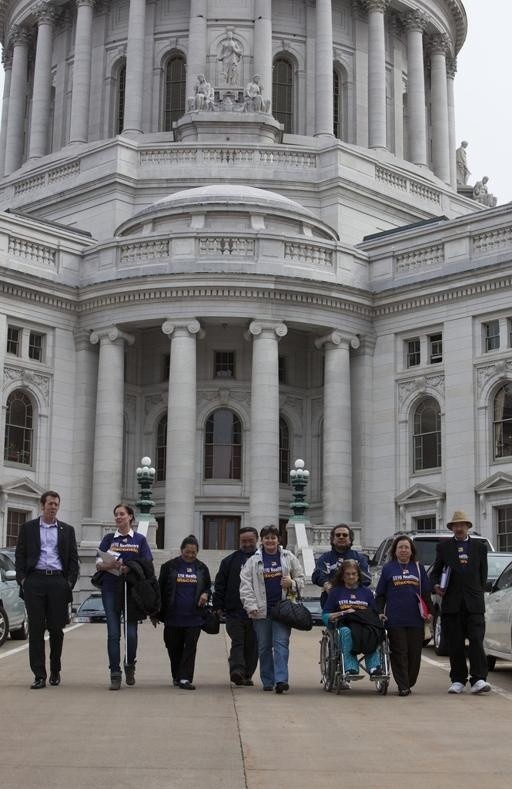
[0,551,30,646]
[413,547,512,673]
[297,596,324,623]
[73,590,110,622]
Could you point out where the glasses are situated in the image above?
[335,533,349,537]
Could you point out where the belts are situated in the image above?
[29,570,63,576]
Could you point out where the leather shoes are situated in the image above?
[31,677,45,688]
[50,671,60,685]
[231,671,253,686]
[263,683,289,693]
[173,678,195,690]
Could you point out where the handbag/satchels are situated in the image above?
[277,599,312,630]
[415,592,430,619]
[92,571,104,590]
[201,605,219,634]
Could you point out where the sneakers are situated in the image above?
[448,682,465,694]
[471,679,491,693]
[400,688,411,695]
[346,669,383,681]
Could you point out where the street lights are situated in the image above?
[286,458,311,524]
[132,456,157,522]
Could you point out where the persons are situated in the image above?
[217,30,243,86]
[15,490,80,690]
[185,73,213,114]
[320,558,389,676]
[456,141,471,185]
[376,535,434,697]
[311,523,370,690]
[237,525,306,694]
[242,74,271,113]
[152,534,212,690]
[94,502,155,690]
[213,527,259,687]
[474,176,489,196]
[430,509,493,695]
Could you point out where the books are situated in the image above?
[96,548,124,577]
[439,573,448,592]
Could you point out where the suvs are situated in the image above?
[358,528,498,600]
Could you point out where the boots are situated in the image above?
[124,660,135,685]
[110,672,121,690]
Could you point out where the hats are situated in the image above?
[447,512,472,531]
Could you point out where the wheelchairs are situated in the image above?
[318,586,393,697]
